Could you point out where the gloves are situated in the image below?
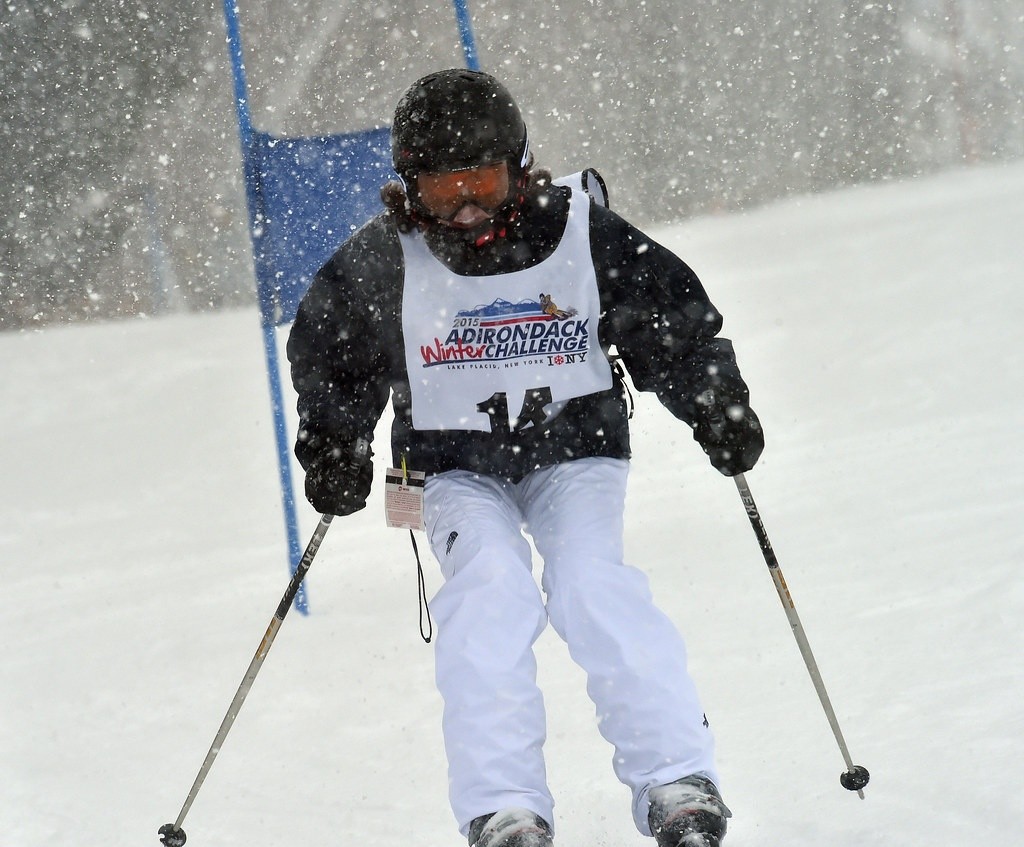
[693,403,766,477]
[305,446,375,516]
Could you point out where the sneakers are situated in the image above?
[468,811,555,847]
[647,775,732,847]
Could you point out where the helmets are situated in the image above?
[391,70,535,267]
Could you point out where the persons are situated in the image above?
[286,68,765,847]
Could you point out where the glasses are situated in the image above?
[406,158,516,221]
